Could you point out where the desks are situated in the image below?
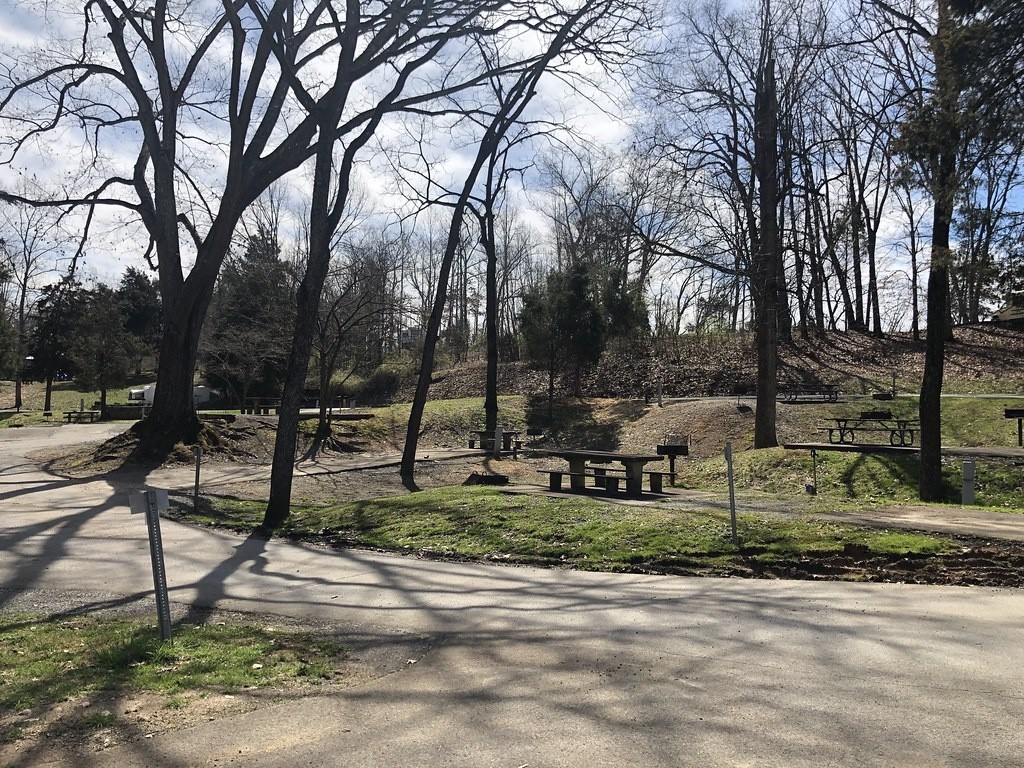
[63,411,99,423]
[823,417,920,447]
[241,397,281,414]
[469,430,521,449]
[546,449,664,495]
[784,382,840,401]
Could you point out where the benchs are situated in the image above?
[63,415,99,423]
[501,440,526,449]
[537,470,633,498]
[469,439,495,449]
[584,466,678,493]
[817,427,921,432]
[783,388,840,393]
[241,405,256,414]
[256,405,281,414]
